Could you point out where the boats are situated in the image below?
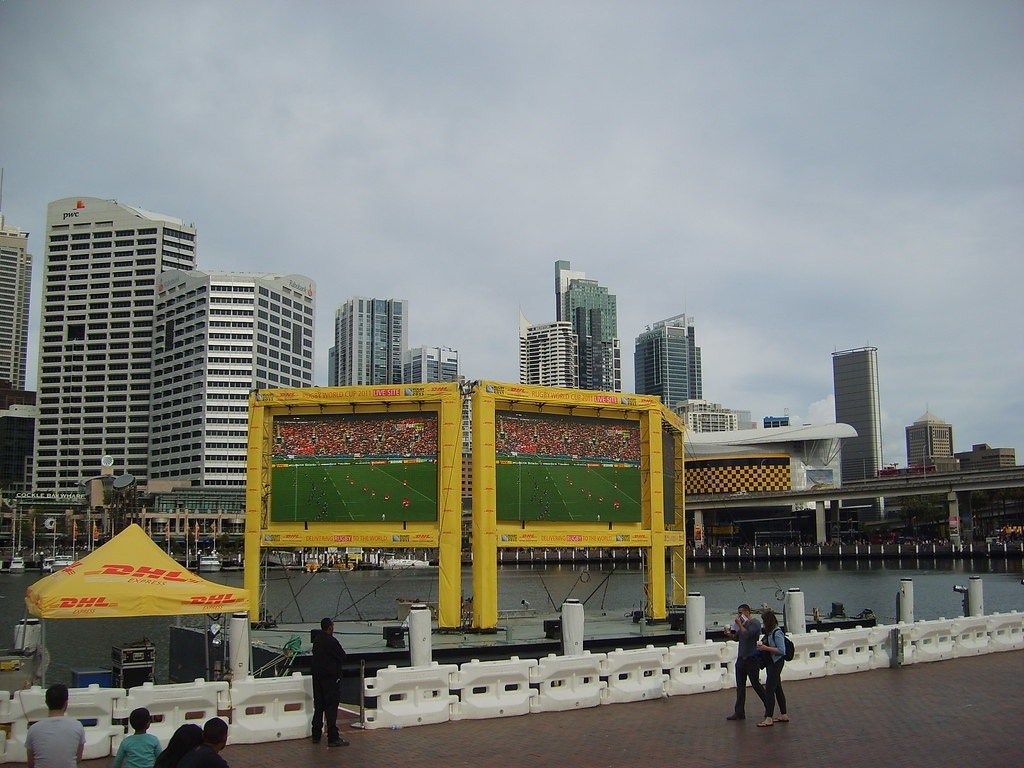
[2,537,434,580]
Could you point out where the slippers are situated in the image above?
[757,720,773,727]
[772,715,790,722]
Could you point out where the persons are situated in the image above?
[268,410,643,524]
[153,724,204,768]
[311,617,350,747]
[724,605,767,721]
[757,611,791,727]
[682,514,1023,566]
[24,685,87,768]
[111,707,162,768]
[10,544,397,562]
[179,717,234,768]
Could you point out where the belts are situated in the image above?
[737,654,759,660]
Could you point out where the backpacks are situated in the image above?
[772,629,794,661]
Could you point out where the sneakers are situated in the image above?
[329,737,349,747]
[312,736,320,744]
[727,711,745,720]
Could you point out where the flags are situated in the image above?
[5,512,221,544]
